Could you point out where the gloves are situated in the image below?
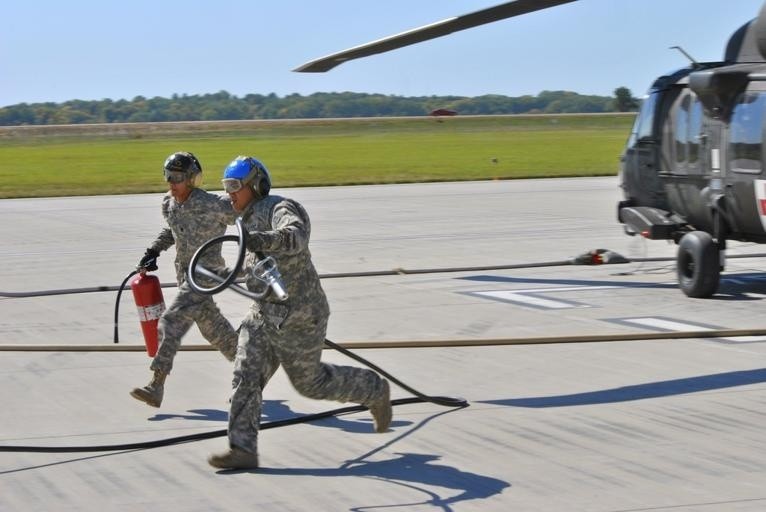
[197,264,230,285]
[246,230,278,253]
[139,248,160,272]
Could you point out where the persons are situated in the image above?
[129,151,239,409]
[207,155,392,469]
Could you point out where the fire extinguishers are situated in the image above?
[114,258,166,357]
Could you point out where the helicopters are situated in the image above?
[291,0,764,310]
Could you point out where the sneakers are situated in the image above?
[370,379,393,433]
[207,446,258,469]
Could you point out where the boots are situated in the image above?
[130,369,167,408]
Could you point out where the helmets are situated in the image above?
[163,150,203,188]
[221,156,272,200]
